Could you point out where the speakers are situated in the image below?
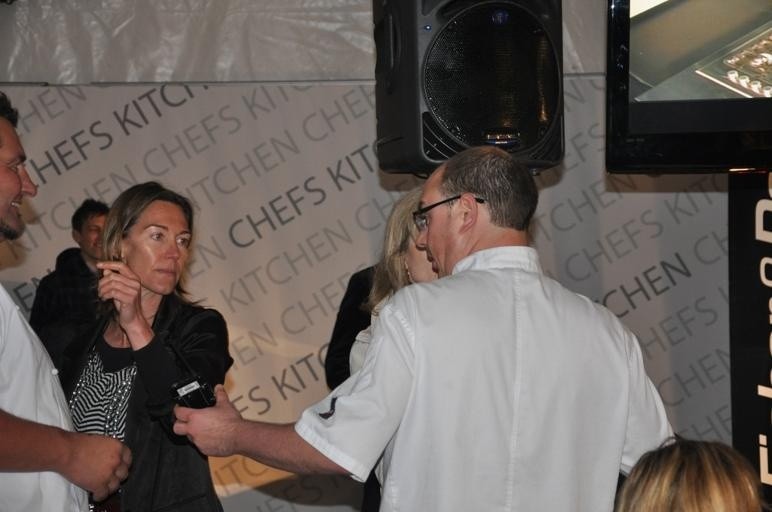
[372,0,565,177]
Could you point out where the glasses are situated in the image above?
[412,195,485,232]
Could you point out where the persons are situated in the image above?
[67,182,234,512]
[30,195,113,399]
[613,433,765,512]
[325,185,439,511]
[171,142,675,511]
[0,86,130,512]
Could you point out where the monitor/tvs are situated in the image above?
[604,0,771,176]
